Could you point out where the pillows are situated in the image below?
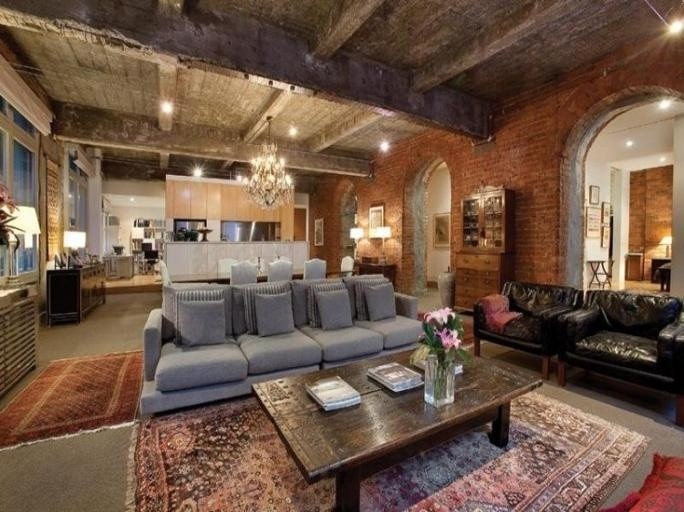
[304,273,397,332]
[602,451,683,512]
[172,288,225,345]
[175,296,228,349]
[250,289,297,339]
[241,280,292,337]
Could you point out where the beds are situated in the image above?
[654,261,671,290]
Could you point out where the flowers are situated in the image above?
[0,181,24,255]
[416,304,472,399]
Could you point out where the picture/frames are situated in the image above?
[432,211,452,248]
[588,183,601,204]
[583,205,603,236]
[366,203,386,240]
[313,217,327,249]
[601,227,612,249]
[599,201,614,224]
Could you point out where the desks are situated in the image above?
[170,268,357,285]
[587,257,614,290]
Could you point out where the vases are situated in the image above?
[420,351,457,406]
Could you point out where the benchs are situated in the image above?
[471,279,585,382]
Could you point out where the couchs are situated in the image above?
[132,272,425,418]
[553,287,683,429]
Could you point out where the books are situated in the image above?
[367,362,425,393]
[411,358,464,375]
[305,376,362,411]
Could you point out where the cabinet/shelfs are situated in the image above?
[45,261,109,331]
[0,283,42,400]
[356,260,397,286]
[451,249,514,312]
[651,258,670,282]
[455,180,516,255]
[100,216,313,286]
[625,250,643,281]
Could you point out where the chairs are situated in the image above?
[673,331,684,425]
[299,257,328,282]
[339,254,356,278]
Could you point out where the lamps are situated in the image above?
[0,202,41,290]
[370,225,394,264]
[63,228,85,261]
[244,113,296,213]
[349,227,365,261]
[657,236,673,259]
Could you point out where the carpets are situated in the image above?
[0,349,145,450]
[124,387,653,510]
